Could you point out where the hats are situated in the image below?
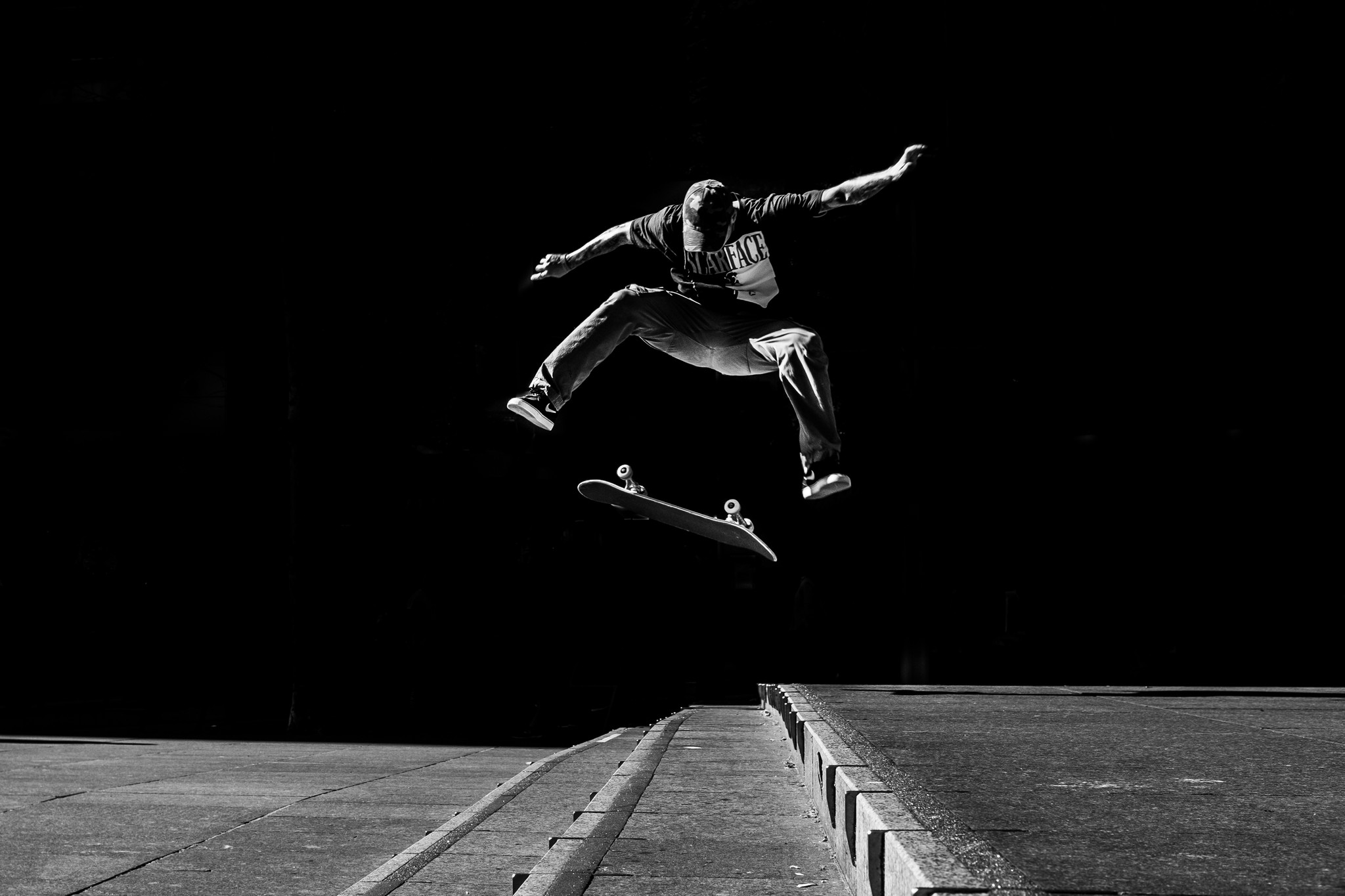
[682,178,733,252]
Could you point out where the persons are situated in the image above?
[505,145,925,499]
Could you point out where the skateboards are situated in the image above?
[577,463,778,564]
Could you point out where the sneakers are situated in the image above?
[506,389,557,431]
[802,460,851,501]
